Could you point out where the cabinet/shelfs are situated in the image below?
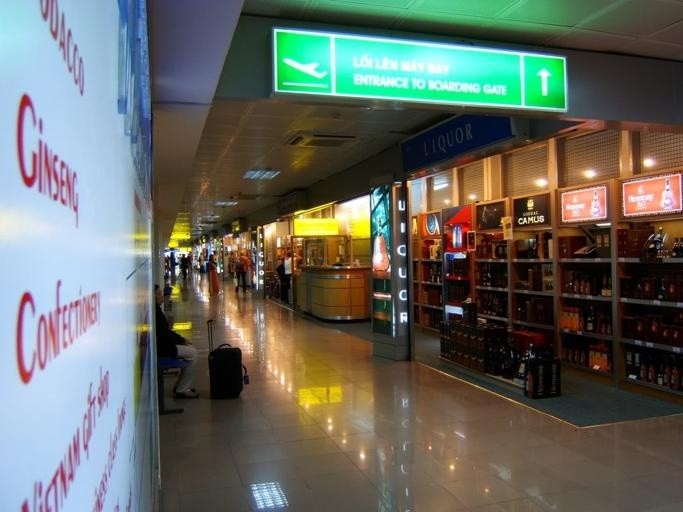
[408,167,683,405]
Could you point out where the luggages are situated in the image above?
[206,319,243,400]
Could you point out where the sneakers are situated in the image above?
[172,386,199,398]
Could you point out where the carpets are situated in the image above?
[269,296,682,429]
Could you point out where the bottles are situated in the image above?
[635,318,683,346]
[523,343,561,399]
[561,307,612,333]
[624,352,683,391]
[437,321,533,381]
[572,271,611,295]
[671,238,683,257]
[648,229,663,260]
[476,289,554,324]
[481,261,494,288]
[566,342,614,373]
[637,277,683,302]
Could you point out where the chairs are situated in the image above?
[157,346,188,415]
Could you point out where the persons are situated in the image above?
[155,284,200,398]
[199,248,218,273]
[277,249,303,304]
[170,251,193,280]
[235,249,248,294]
[208,254,220,297]
